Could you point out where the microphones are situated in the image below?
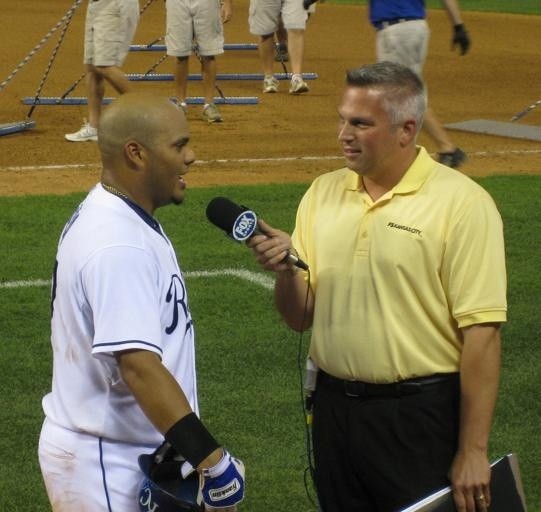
[206,197,309,271]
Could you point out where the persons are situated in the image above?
[248,0,317,95]
[165,0,232,123]
[64,0,140,143]
[303,0,472,168]
[246,62,507,512]
[39,89,246,512]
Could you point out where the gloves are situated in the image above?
[194,448,248,512]
[448,23,473,57]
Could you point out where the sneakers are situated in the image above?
[201,104,223,123]
[63,123,99,145]
[435,148,469,170]
[259,72,281,98]
[288,72,308,96]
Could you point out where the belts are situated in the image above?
[308,367,460,400]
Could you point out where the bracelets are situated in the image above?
[165,412,221,468]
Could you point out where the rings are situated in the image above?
[474,494,485,500]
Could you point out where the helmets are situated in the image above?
[135,433,204,512]
